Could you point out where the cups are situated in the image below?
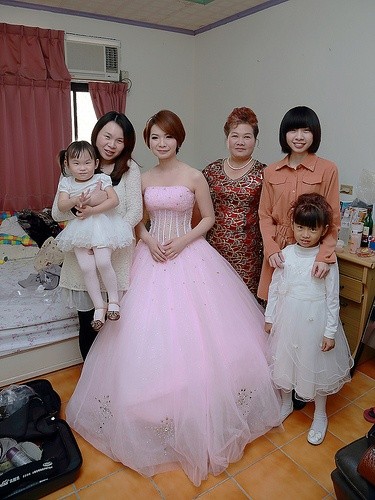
[350,222,364,247]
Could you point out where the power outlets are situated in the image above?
[340,185,353,195]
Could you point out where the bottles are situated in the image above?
[360,208,373,248]
[6,447,32,467]
[339,208,350,246]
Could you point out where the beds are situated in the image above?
[0,210,85,388]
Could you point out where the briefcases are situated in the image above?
[0,378,83,500]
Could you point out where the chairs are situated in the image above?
[330,435,375,500]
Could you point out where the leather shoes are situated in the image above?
[91,307,107,331]
[106,302,121,320]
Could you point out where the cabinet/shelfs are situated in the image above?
[334,247,375,359]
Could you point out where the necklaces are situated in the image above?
[227,157,253,170]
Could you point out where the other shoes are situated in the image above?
[272,402,293,427]
[307,416,327,445]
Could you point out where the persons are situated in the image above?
[257,106,342,300]
[264,191,354,444]
[55,140,135,330]
[51,112,143,359]
[203,107,268,307]
[65,109,285,487]
